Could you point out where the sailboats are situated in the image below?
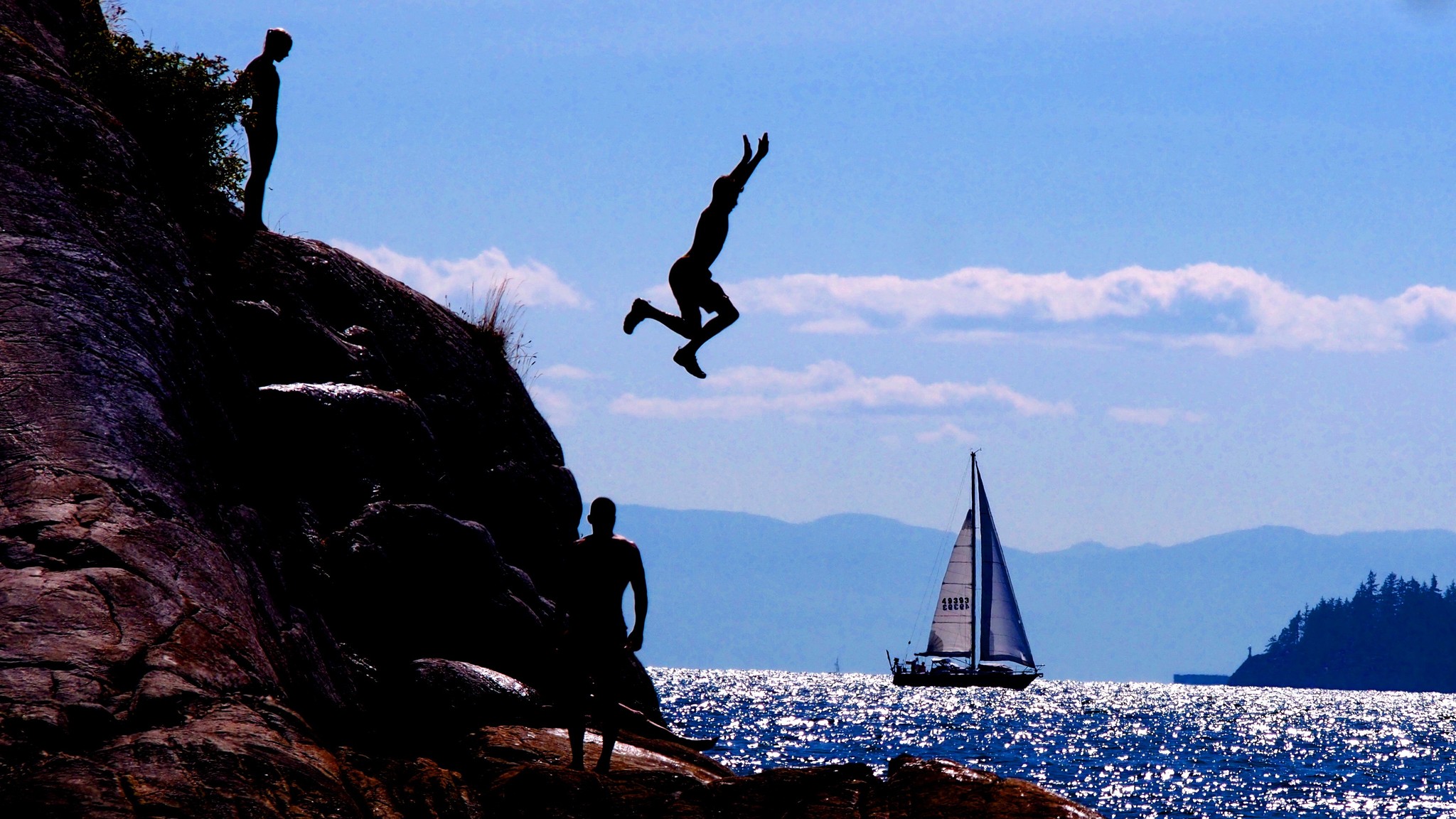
[885,451,1046,689]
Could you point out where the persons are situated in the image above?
[232,28,293,231]
[622,132,771,380]
[548,493,650,774]
